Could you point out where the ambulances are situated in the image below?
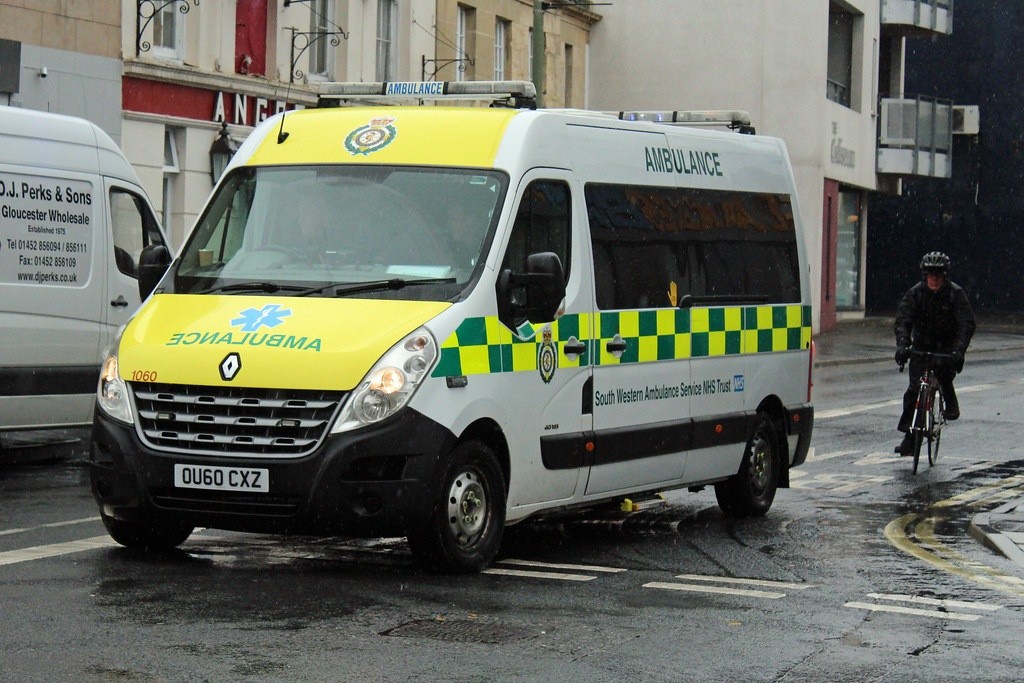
[88,81,817,579]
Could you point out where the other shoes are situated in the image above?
[943,401,960,420]
[895,433,916,455]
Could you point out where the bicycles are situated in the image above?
[898,344,958,475]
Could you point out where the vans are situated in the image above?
[0,105,174,448]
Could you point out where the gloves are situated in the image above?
[895,346,909,366]
[944,352,965,374]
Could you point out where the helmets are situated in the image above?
[919,251,951,274]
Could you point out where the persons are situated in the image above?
[438,193,509,268]
[893,251,977,452]
[296,191,353,267]
